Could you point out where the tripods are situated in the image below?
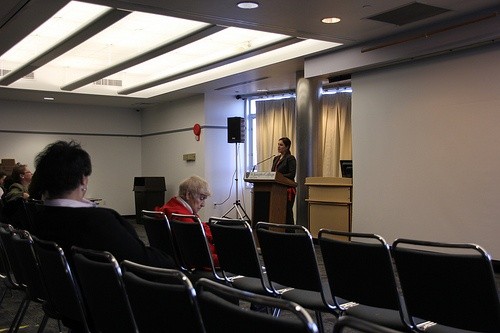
[221,143,250,220]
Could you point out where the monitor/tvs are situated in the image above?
[340,160,353,178]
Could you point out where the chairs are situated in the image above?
[0,195,500,333]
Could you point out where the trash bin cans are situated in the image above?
[132,177,167,225]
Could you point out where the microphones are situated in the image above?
[253,155,275,172]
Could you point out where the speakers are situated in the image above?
[227,116,246,143]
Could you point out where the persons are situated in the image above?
[0,165,32,202]
[271,138,296,234]
[156,175,219,268]
[28,141,169,281]
